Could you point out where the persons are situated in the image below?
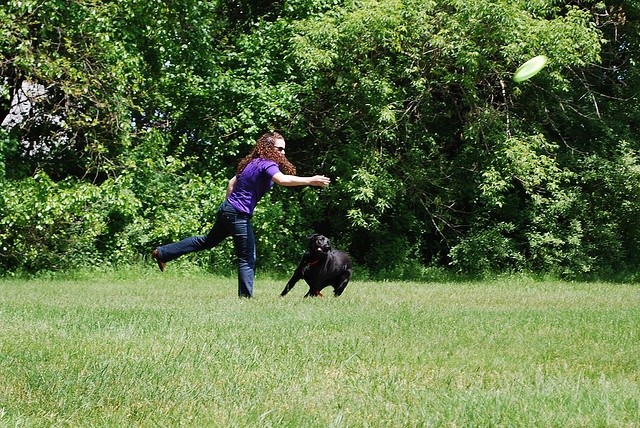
[152,132,332,297]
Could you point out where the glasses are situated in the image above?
[275,146,285,151]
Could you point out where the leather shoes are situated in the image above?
[153,249,164,272]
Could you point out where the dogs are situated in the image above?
[279,233,353,299]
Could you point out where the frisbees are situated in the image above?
[513,54,548,82]
